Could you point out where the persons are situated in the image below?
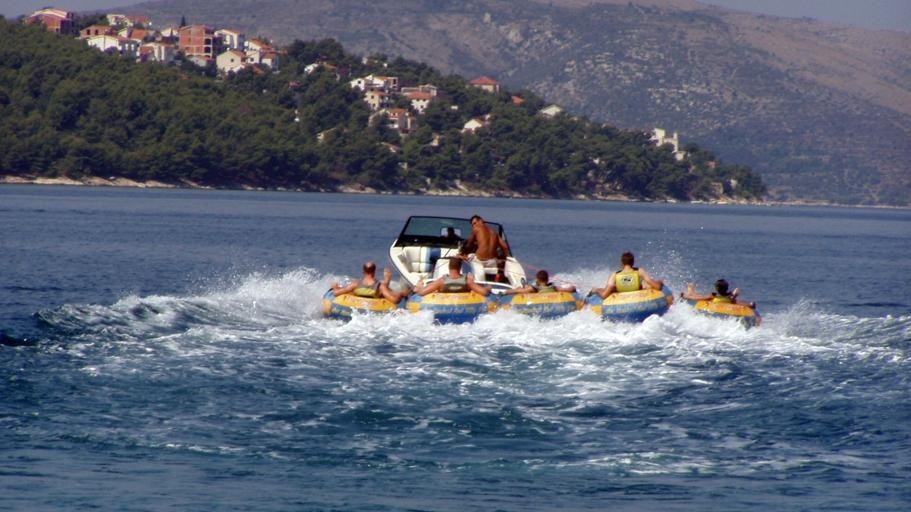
[681,277,740,303]
[330,261,408,304]
[418,257,491,297]
[504,270,576,295]
[446,226,463,246]
[465,215,511,267]
[589,253,664,300]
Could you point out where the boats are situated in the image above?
[326,215,527,324]
[512,280,761,333]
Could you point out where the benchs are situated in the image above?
[400,246,461,276]
